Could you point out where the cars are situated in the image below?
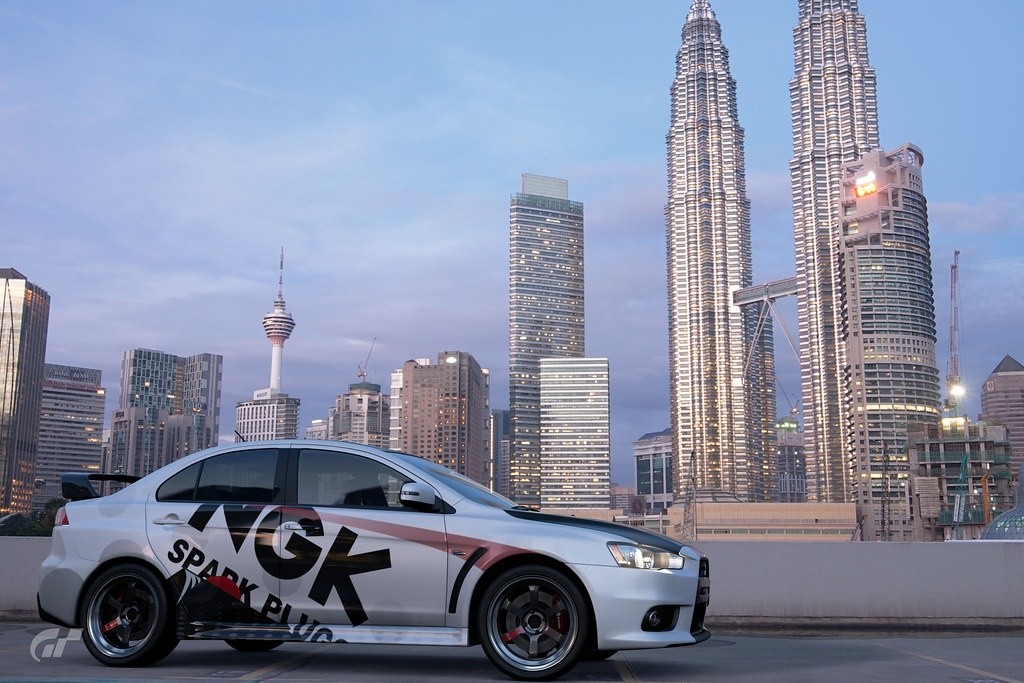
[36,437,713,682]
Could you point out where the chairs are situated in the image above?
[342,480,365,506]
[300,476,320,504]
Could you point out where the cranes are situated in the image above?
[947,457,970,540]
[982,469,994,525]
